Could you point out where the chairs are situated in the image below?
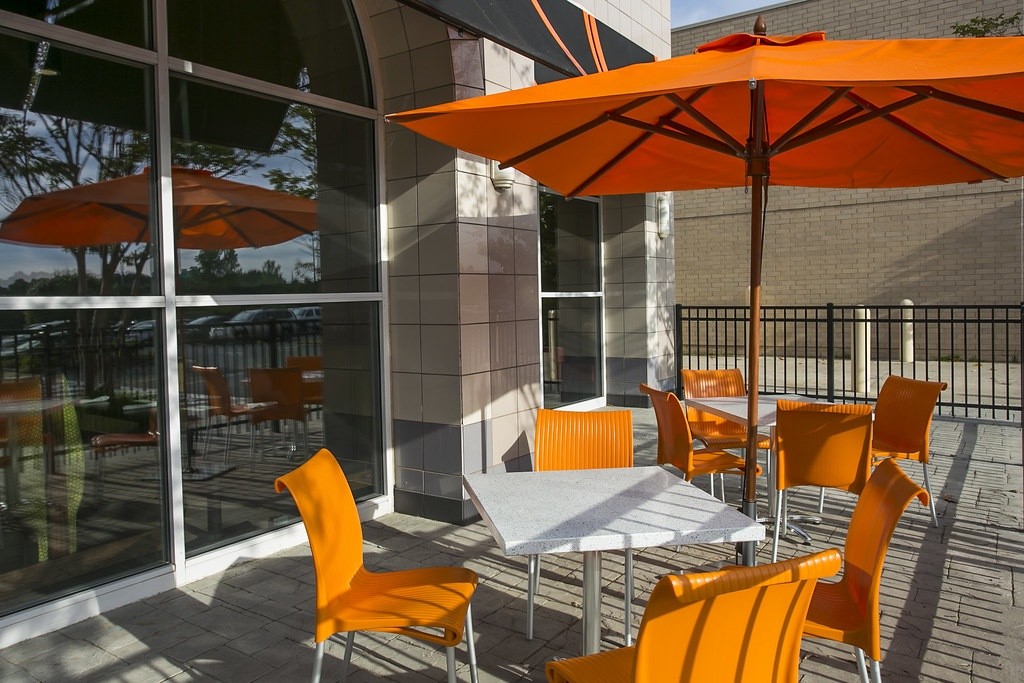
[0,356,324,503]
[275,368,948,683]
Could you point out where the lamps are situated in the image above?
[490,160,515,188]
[657,196,669,238]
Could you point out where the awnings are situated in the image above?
[395,0,659,78]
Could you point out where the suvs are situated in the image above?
[208,307,299,342]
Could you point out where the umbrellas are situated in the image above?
[0,167,319,427]
[383,14,1023,567]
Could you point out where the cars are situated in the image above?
[121,319,158,347]
[0,320,120,369]
[179,314,229,342]
[294,307,322,331]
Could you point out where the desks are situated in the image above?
[686,394,835,546]
[463,465,766,656]
